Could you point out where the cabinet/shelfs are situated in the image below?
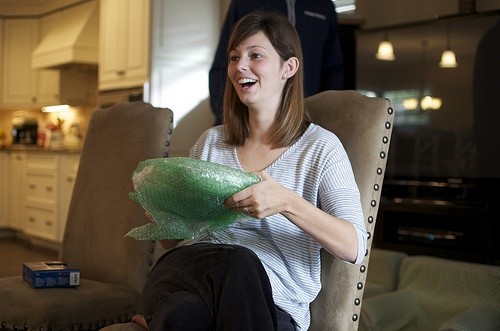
[0,150,81,253]
[99,0,153,92]
[0,16,61,108]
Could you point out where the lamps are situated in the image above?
[374,30,395,61]
[437,22,458,69]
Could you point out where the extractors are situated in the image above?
[30,2,98,109]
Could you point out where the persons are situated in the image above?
[133,12,368,331]
[208,0,346,100]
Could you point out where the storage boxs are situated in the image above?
[22,262,81,289]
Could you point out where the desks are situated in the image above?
[374,197,500,264]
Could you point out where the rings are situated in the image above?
[243,207,250,214]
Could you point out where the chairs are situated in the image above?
[301,89,395,331]
[99,97,226,331]
[0,100,174,331]
